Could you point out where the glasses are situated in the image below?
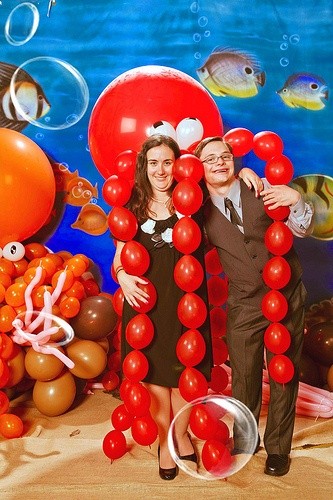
[201,153,234,165]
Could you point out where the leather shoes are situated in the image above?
[264,451,289,476]
[232,448,251,455]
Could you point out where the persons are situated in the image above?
[112,134,264,480]
[194,137,313,478]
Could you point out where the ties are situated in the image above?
[224,197,242,228]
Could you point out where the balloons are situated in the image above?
[225,127,294,385]
[0,243,124,439]
[103,148,231,476]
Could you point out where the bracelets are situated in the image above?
[117,269,124,274]
[116,266,123,271]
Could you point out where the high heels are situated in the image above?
[158,443,175,480]
[175,433,197,463]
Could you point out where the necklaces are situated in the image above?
[152,196,170,208]
